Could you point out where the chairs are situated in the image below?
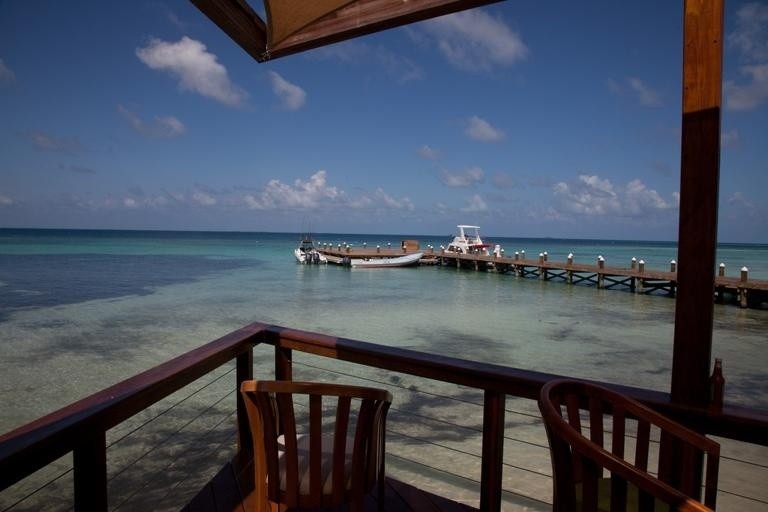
[232,379,394,512]
[532,376,724,512]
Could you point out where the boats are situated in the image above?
[444,224,494,271]
[337,252,425,269]
[293,231,329,265]
